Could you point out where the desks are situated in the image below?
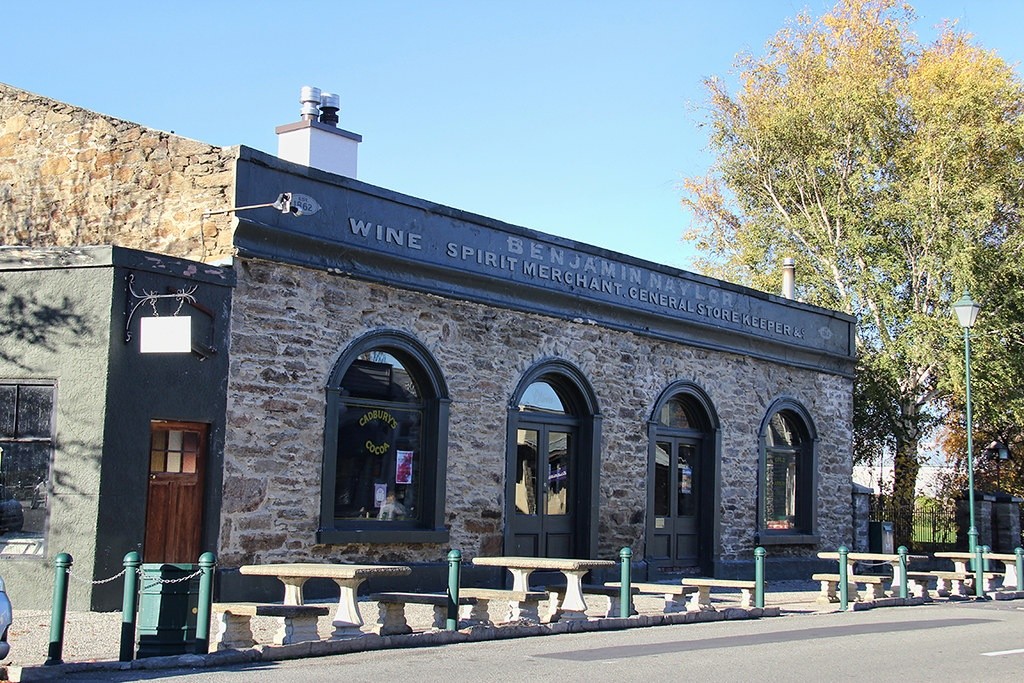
[817,552,928,597]
[472,556,616,623]
[239,563,411,640]
[934,552,1018,587]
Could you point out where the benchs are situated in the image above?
[930,571,974,600]
[447,588,549,624]
[212,605,329,650]
[813,574,892,603]
[370,592,476,637]
[906,575,937,602]
[546,583,639,623]
[605,582,700,613]
[682,578,767,608]
[971,573,1005,591]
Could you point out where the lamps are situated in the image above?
[235,191,303,217]
